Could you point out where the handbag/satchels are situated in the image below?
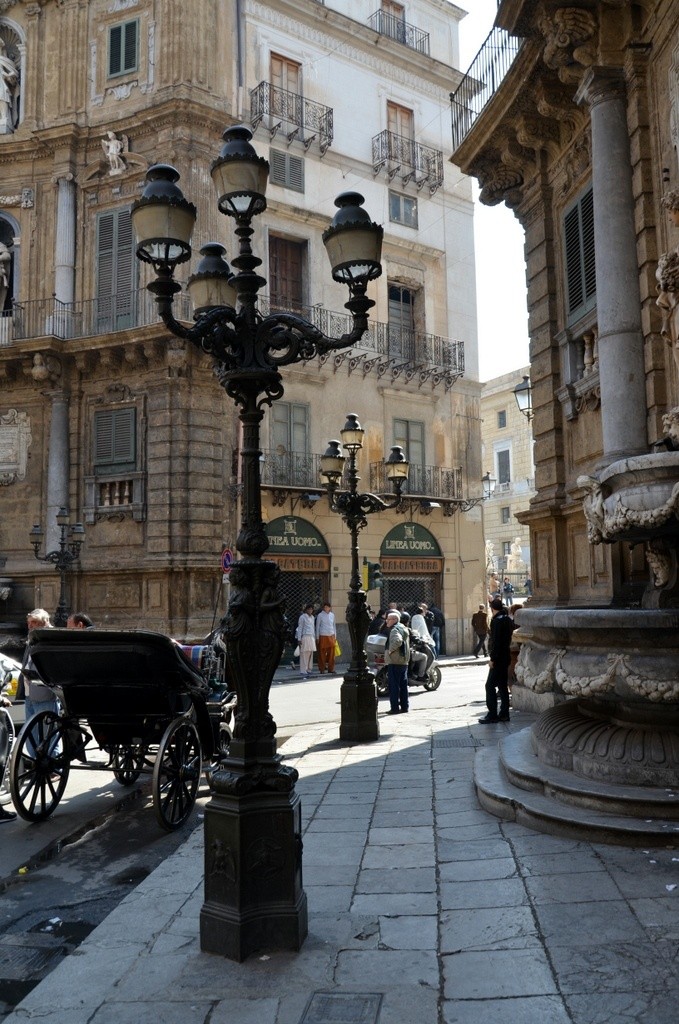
[334,640,340,657]
[433,615,444,626]
[294,645,300,657]
[29,680,55,702]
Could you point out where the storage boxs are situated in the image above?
[366,635,387,654]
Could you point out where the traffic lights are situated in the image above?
[368,562,383,590]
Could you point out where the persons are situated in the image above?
[369,610,375,619]
[0,37,19,120]
[471,604,489,658]
[298,605,317,675]
[316,602,336,674]
[345,595,358,651]
[655,187,679,372]
[291,604,307,670]
[0,242,11,317]
[537,15,555,63]
[577,475,603,530]
[511,537,522,569]
[220,568,254,722]
[478,599,523,723]
[15,609,61,784]
[0,657,18,823]
[359,593,372,651]
[384,613,410,714]
[67,613,93,628]
[256,563,286,720]
[368,609,386,635]
[487,572,533,694]
[645,550,673,586]
[388,599,445,658]
[486,539,494,568]
[101,130,121,170]
[32,352,48,380]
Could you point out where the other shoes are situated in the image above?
[51,772,61,782]
[417,676,430,681]
[386,709,400,714]
[479,712,499,723]
[472,651,479,658]
[400,708,408,713]
[0,808,17,823]
[499,711,510,721]
[486,655,488,657]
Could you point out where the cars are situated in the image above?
[0,652,65,727]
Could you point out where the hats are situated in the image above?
[490,599,503,610]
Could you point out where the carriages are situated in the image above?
[8,623,238,834]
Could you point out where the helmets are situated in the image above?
[387,609,401,616]
[401,612,411,619]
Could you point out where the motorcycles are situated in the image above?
[365,633,442,698]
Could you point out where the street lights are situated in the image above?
[320,412,409,742]
[131,121,386,964]
[29,505,85,627]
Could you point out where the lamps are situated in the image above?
[509,375,533,424]
[301,495,321,508]
[421,502,443,508]
[459,471,497,512]
